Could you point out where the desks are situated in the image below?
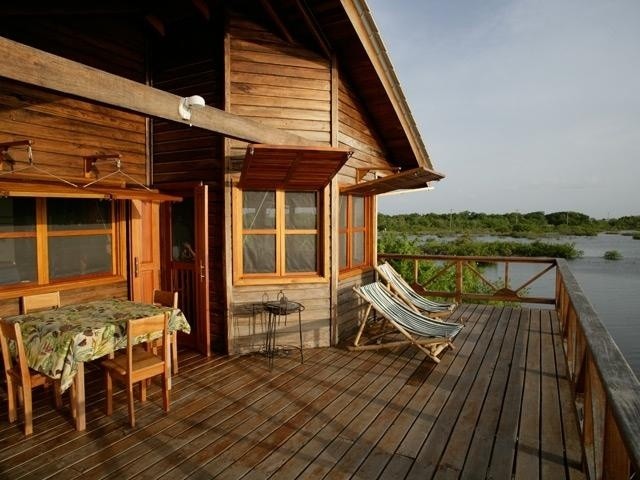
[253,297,306,375]
[4,297,176,432]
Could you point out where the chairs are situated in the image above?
[99,308,170,430]
[139,288,182,376]
[370,258,460,346]
[0,318,64,440]
[346,279,464,365]
[18,287,63,395]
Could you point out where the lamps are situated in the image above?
[180,94,206,120]
